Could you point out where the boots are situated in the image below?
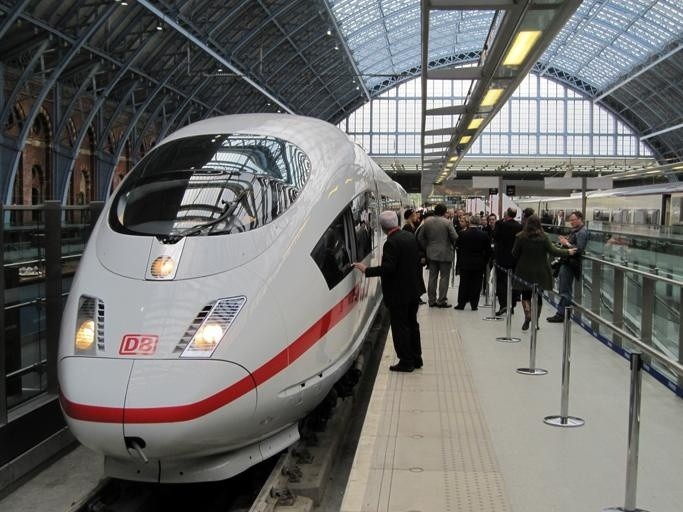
[520,299,533,330]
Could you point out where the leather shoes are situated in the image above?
[494,307,508,316]
[389,358,423,373]
[429,301,479,311]
[547,312,570,323]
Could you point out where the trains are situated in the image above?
[514,181,683,276]
[56,113,414,485]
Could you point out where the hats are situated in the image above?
[403,209,415,219]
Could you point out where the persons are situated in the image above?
[350,210,426,372]
[546,210,589,323]
[401,201,578,330]
